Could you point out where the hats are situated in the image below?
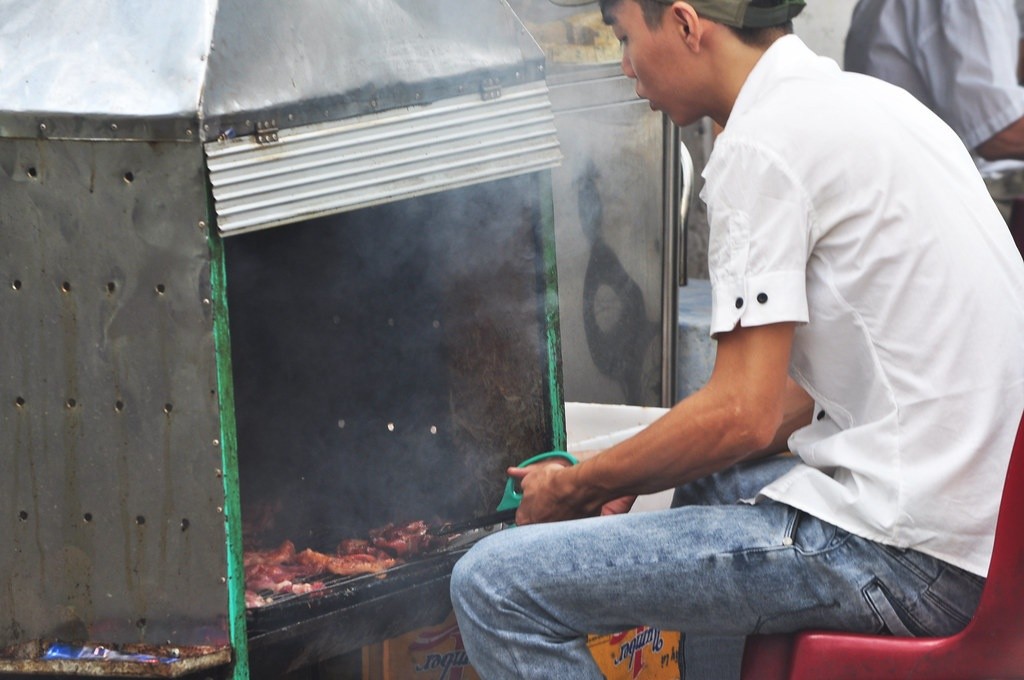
[549,0,807,28]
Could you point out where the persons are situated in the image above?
[450,0,1024,680]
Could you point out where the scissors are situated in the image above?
[429,449,580,557]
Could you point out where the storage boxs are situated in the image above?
[354,400,681,680]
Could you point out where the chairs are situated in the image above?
[740,414,1023,680]
